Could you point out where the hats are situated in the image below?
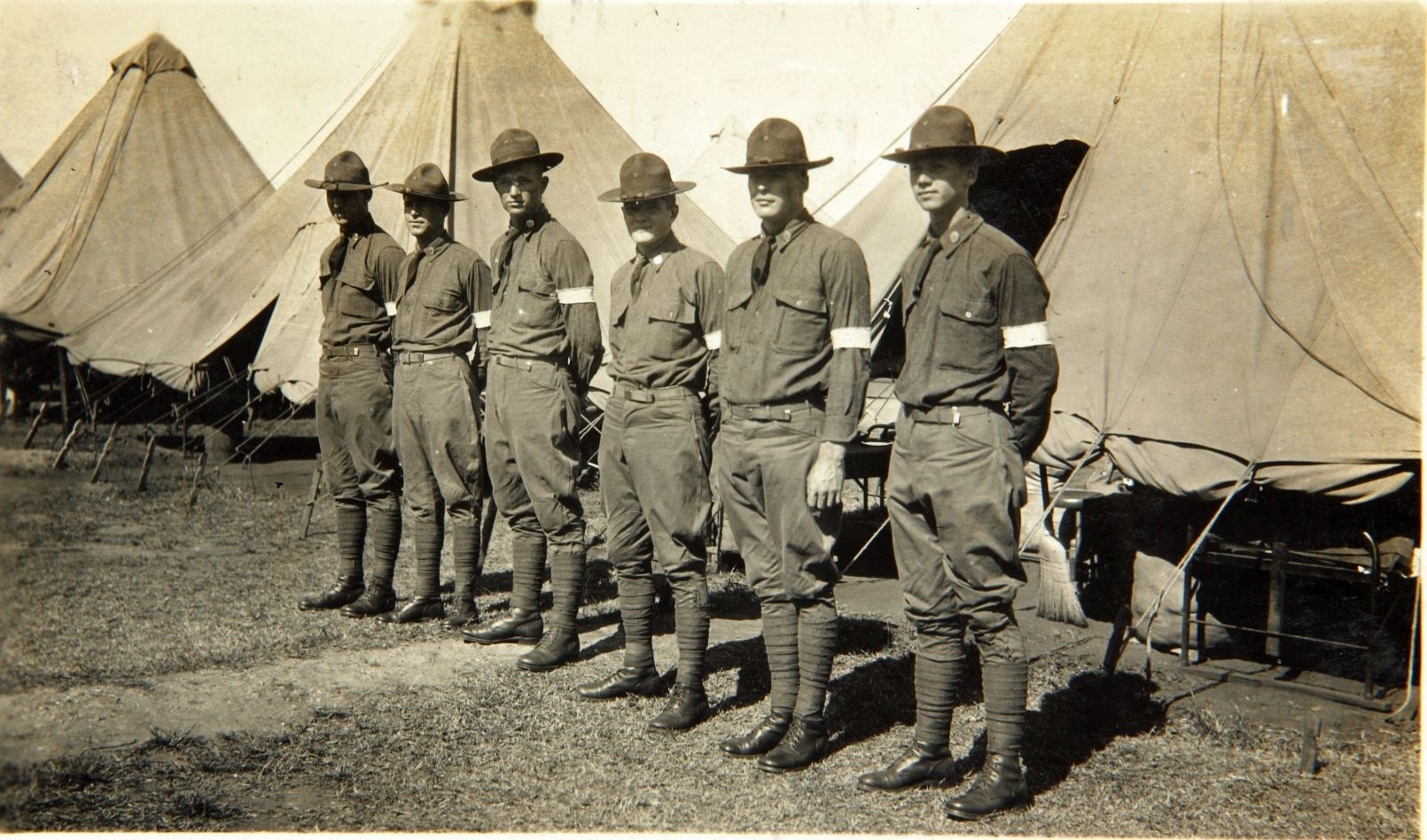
[725,120,832,173]
[883,105,1008,164]
[596,153,696,203]
[384,163,468,201]
[471,129,563,181]
[304,152,389,191]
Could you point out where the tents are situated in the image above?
[19,0,738,536]
[0,32,278,423]
[813,3,1427,724]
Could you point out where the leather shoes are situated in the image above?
[857,740,956,791]
[296,577,367,611]
[460,609,543,645]
[758,717,826,771]
[517,632,581,670]
[720,715,795,758]
[648,684,710,733]
[577,668,664,702]
[948,754,1031,822]
[340,577,398,617]
[376,598,444,624]
[439,598,478,630]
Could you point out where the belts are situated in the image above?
[322,343,387,356]
[614,383,697,403]
[489,352,570,371]
[395,346,459,364]
[729,400,823,421]
[904,402,1004,426]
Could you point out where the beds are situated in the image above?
[1179,500,1415,700]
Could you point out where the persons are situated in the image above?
[376,162,491,630]
[856,106,1060,820]
[719,117,872,773]
[460,130,606,672]
[578,153,728,732]
[298,151,410,618]
